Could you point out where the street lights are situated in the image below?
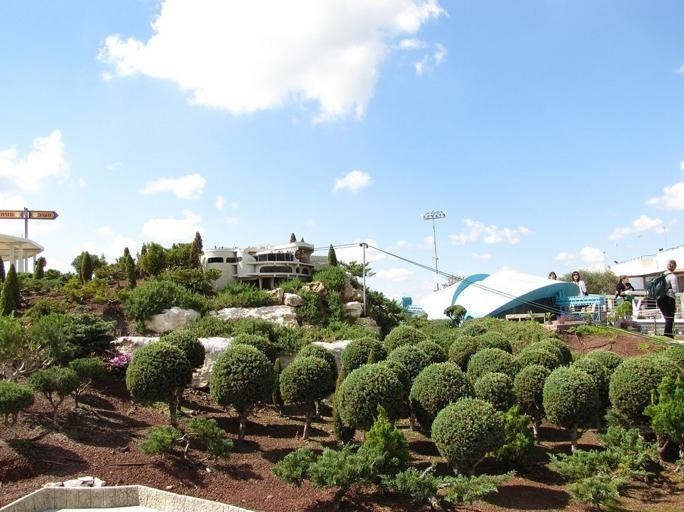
[420,209,449,289]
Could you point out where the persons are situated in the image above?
[655,259,679,338]
[548,271,556,281]
[569,271,589,314]
[615,274,634,299]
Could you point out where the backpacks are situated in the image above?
[647,270,674,300]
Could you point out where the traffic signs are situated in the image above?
[0,210,58,220]
